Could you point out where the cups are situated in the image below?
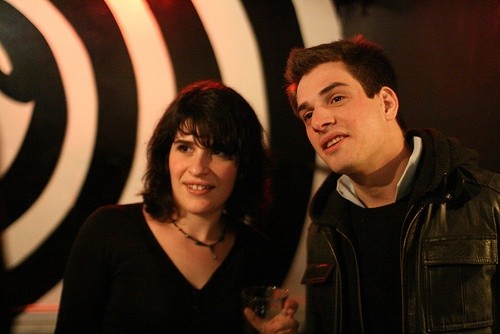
[243,285,289,334]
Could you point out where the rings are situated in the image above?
[283,302,295,314]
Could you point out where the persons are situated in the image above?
[287,38,500,334]
[53,82,299,334]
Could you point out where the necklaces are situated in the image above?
[167,217,226,259]
